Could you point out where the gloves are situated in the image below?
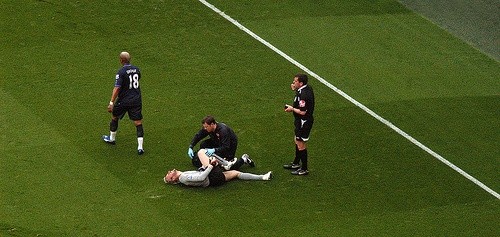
[188,148,194,159]
[205,148,215,156]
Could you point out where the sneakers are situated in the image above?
[283,163,300,170]
[242,154,255,167]
[291,168,308,175]
[138,149,143,155]
[103,135,115,145]
[263,171,272,181]
[224,158,237,170]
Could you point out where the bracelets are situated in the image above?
[109,101,114,105]
[291,108,294,111]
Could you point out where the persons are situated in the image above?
[283,74,315,176]
[186,115,237,167]
[164,149,272,187]
[102,52,145,155]
